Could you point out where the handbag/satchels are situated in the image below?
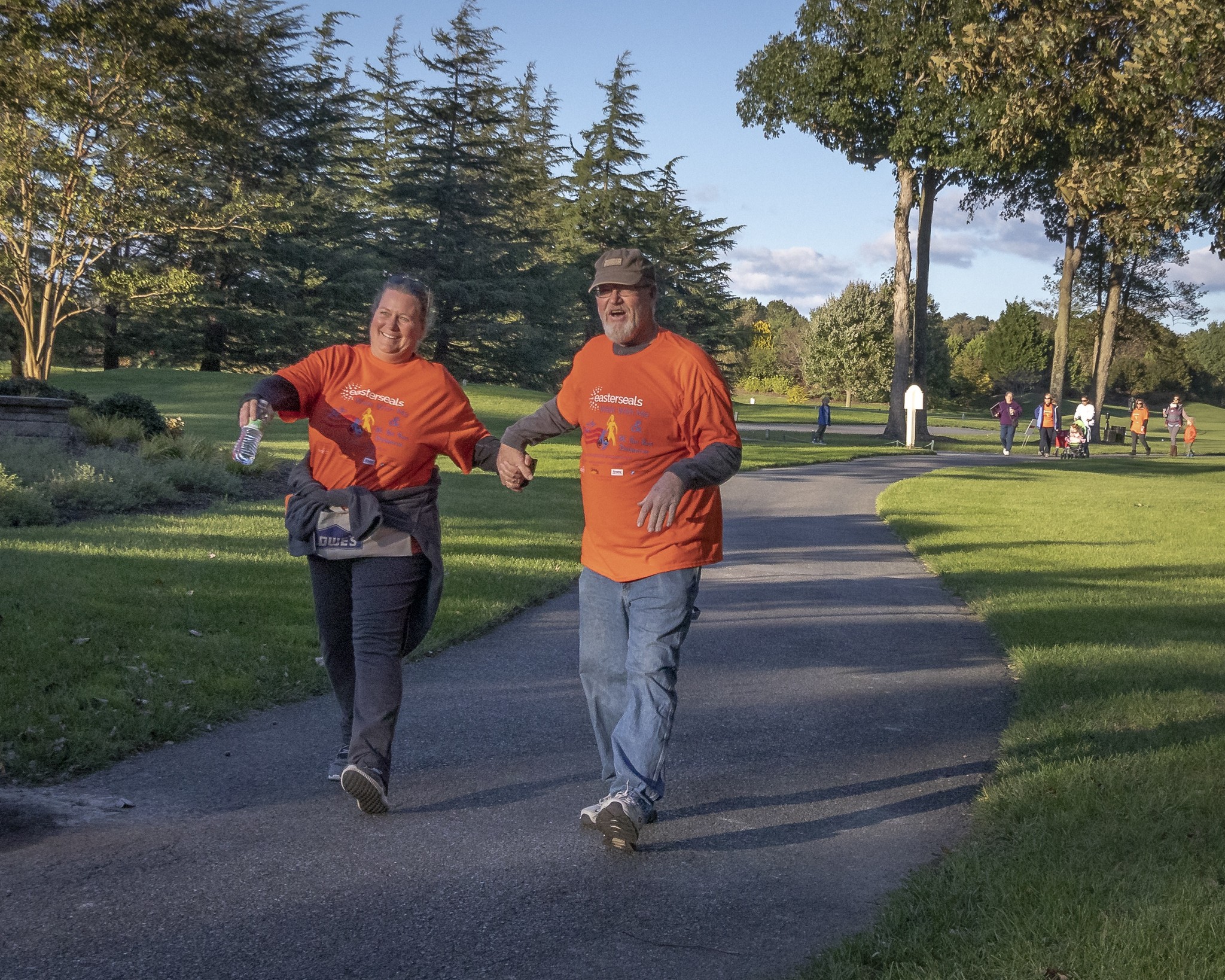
[1011,417,1019,428]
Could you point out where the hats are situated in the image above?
[588,248,658,294]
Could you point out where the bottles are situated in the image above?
[231,398,268,465]
[1009,407,1014,415]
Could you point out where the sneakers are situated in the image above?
[812,437,819,445]
[328,745,349,782]
[578,793,606,827]
[1145,447,1151,455]
[1005,450,1010,455]
[349,763,389,815]
[596,779,642,858]
[1003,448,1007,454]
[817,440,827,445]
[1129,449,1136,456]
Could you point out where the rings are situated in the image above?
[517,479,520,484]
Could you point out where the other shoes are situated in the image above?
[1045,453,1050,458]
[1039,451,1043,456]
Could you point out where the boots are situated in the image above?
[1173,446,1178,457]
[1169,445,1175,456]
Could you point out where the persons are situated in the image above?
[1163,394,1190,457]
[812,396,832,445]
[1129,398,1152,456]
[1069,424,1084,445]
[496,249,743,854]
[995,391,1023,456]
[1073,394,1097,459]
[1034,393,1062,457]
[238,274,537,814]
[1185,416,1198,459]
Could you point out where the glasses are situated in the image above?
[1173,398,1179,401]
[590,284,637,298]
[1136,402,1142,405]
[1044,397,1050,399]
[1080,400,1086,402]
